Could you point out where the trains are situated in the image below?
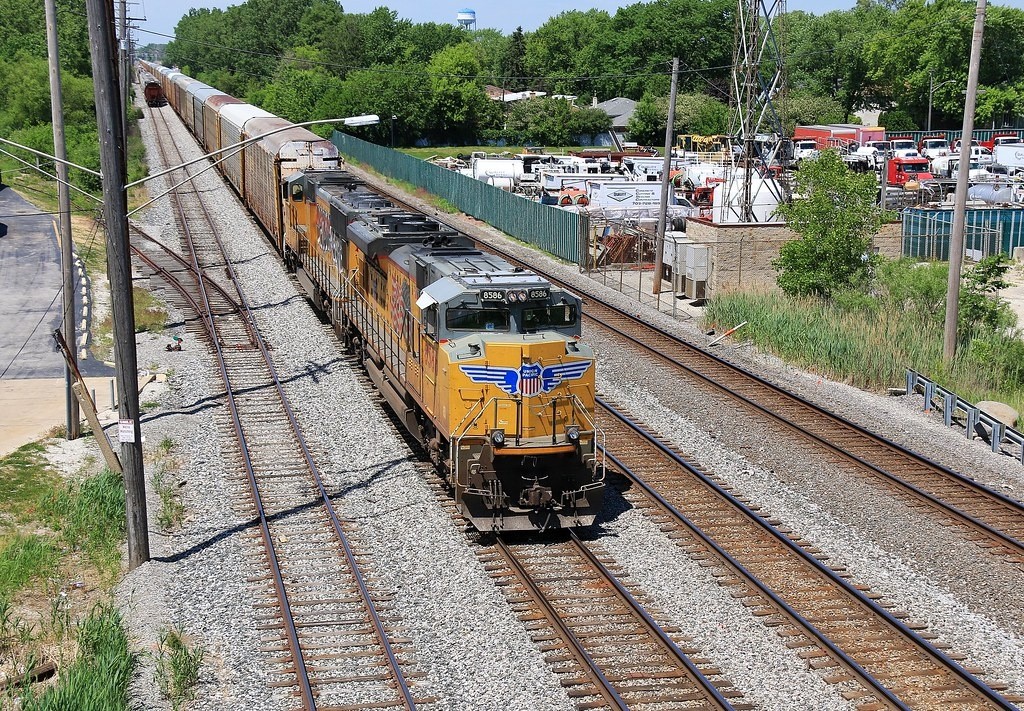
[133,56,609,533]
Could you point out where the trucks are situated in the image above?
[464,132,1024,231]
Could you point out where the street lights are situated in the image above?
[928,72,959,133]
[99,113,383,575]
